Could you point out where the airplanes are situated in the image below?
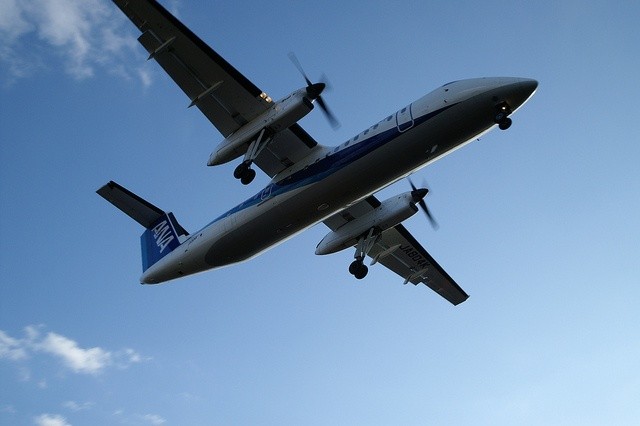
[94,0,539,306]
